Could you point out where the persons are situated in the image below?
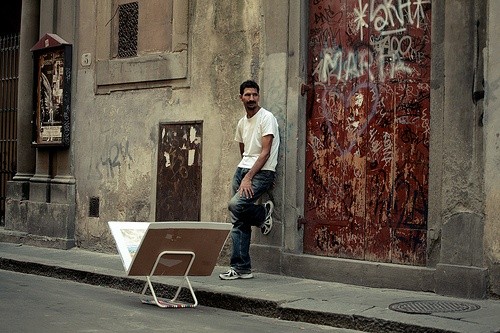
[219,80,280,282]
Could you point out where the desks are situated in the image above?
[108,220,234,307]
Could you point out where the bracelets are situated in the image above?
[245,175,252,179]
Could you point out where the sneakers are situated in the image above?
[219,269,254,279]
[261,200,275,234]
